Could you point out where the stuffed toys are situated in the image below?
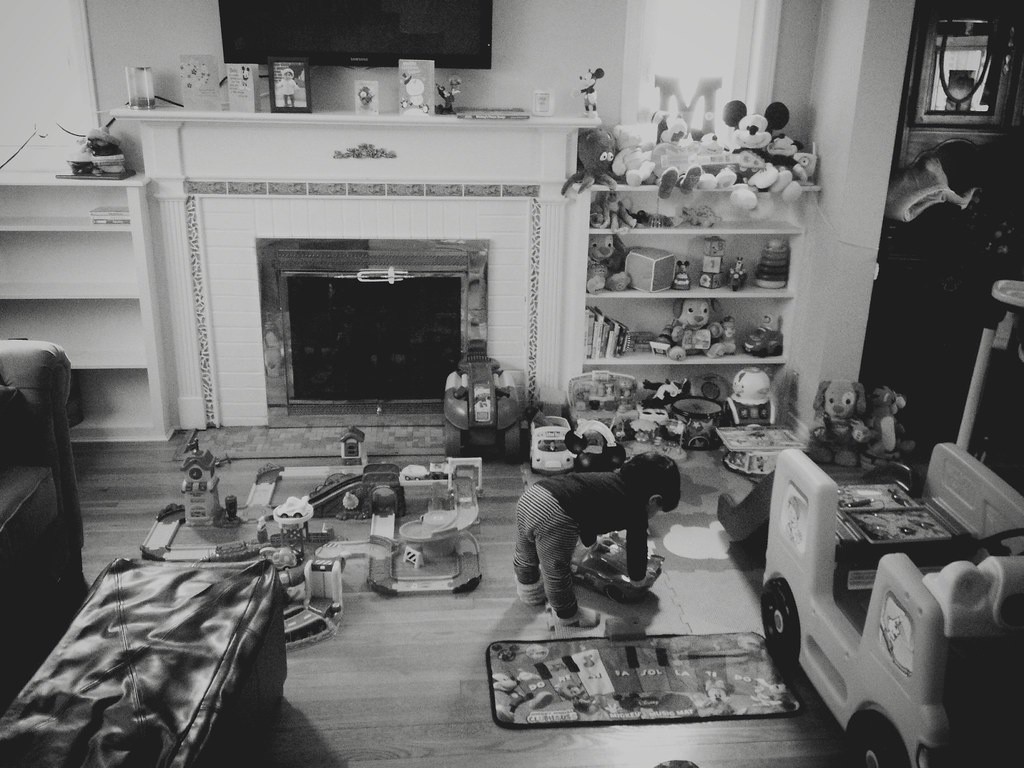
[640,297,915,472]
[561,100,817,292]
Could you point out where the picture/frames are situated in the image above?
[267,54,312,113]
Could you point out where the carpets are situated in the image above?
[485,632,805,730]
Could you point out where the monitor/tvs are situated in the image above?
[219,0,494,70]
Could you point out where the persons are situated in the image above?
[513,453,681,628]
[276,68,299,107]
[548,442,557,452]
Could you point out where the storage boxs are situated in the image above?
[624,247,677,294]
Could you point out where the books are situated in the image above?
[585,305,655,360]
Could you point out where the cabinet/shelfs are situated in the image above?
[0,171,174,443]
[573,183,823,424]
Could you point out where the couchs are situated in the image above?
[0,338,85,719]
[0,556,287,768]
